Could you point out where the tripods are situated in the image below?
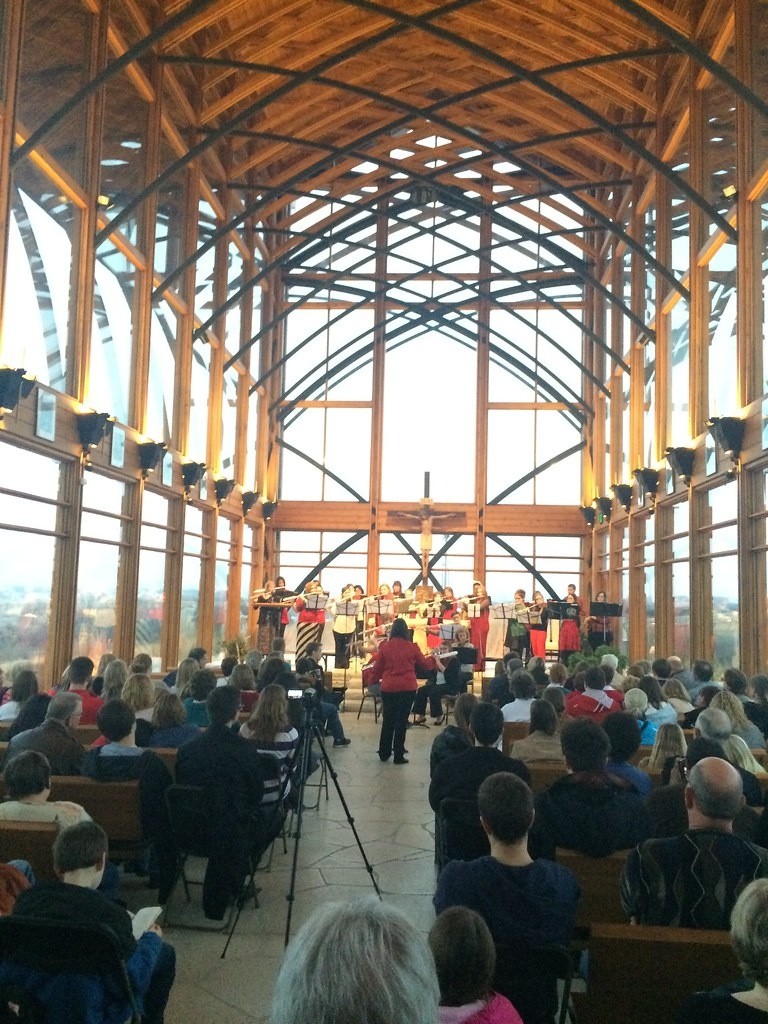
[219,707,383,959]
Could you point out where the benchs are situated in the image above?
[503,722,768,1024]
[0,667,224,885]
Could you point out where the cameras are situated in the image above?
[286,687,319,709]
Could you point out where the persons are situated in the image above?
[0,575,768,1024]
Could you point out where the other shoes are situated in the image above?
[394,757,409,765]
[333,738,350,748]
[404,750,408,753]
[325,730,333,736]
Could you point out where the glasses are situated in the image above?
[691,723,696,729]
[684,767,693,787]
[721,677,727,682]
[72,712,84,720]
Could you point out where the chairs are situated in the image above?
[259,752,289,854]
[163,785,260,932]
[0,914,146,1024]
[442,672,461,727]
[438,798,482,873]
[358,667,385,724]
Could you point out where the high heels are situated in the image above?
[413,718,426,725]
[434,714,445,726]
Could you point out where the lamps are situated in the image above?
[579,507,595,529]
[1,368,36,418]
[664,447,695,487]
[76,413,114,454]
[704,417,745,465]
[263,500,278,520]
[215,479,236,507]
[243,492,260,517]
[139,442,169,478]
[182,462,207,494]
[632,469,658,503]
[610,485,631,514]
[593,497,611,523]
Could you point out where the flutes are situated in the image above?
[250,585,545,614]
[357,622,394,636]
[418,621,458,630]
[438,638,471,651]
[344,637,387,647]
